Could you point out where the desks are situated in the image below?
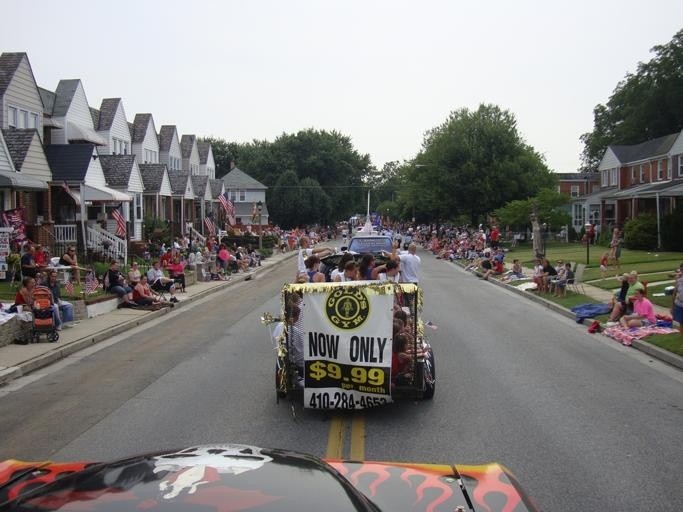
[256,200,264,226]
[583,222,593,264]
[186,218,194,253]
[414,165,428,170]
[541,222,546,255]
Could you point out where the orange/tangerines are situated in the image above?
[27,284,59,344]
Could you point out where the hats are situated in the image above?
[0,443,539,512]
[260,216,439,405]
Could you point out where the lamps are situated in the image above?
[549,260,586,297]
[173,279,183,292]
[51,256,71,282]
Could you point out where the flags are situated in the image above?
[112,211,126,237]
[219,190,237,213]
[82,270,100,296]
[204,217,214,233]
[2,207,26,242]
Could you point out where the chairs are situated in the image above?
[125,300,138,307]
[170,297,179,302]
[174,278,182,283]
[55,325,62,330]
[64,323,77,328]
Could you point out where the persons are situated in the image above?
[102,233,259,308]
[251,223,338,252]
[413,222,504,280]
[513,259,521,278]
[534,255,577,303]
[10,244,81,334]
[275,211,421,384]
[581,215,683,336]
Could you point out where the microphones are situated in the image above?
[154,288,168,302]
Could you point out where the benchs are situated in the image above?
[219,190,237,213]
[2,207,26,242]
[82,270,100,296]
